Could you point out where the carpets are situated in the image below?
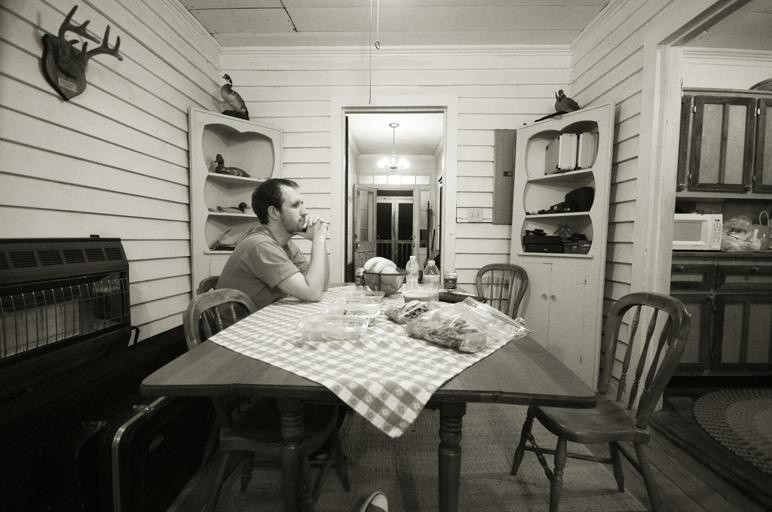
[693,386,772,476]
[201,341,649,512]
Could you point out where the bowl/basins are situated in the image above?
[403,289,433,303]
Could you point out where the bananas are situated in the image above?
[364,257,397,273]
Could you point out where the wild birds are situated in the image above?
[215,153,250,177]
[217,202,251,213]
[220,73,248,114]
[555,89,580,112]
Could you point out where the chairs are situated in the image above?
[510,292,693,512]
[183,288,350,512]
[196,276,223,294]
[475,263,528,321]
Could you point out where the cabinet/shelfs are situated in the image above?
[675,88,772,203]
[189,107,283,300]
[669,251,772,385]
[507,102,617,393]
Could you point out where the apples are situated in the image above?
[364,268,401,294]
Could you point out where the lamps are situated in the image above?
[377,122,409,174]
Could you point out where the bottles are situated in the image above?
[444,267,458,292]
[406,256,419,285]
[423,260,440,285]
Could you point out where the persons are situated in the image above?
[212,178,346,468]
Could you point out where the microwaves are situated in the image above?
[672,214,723,250]
